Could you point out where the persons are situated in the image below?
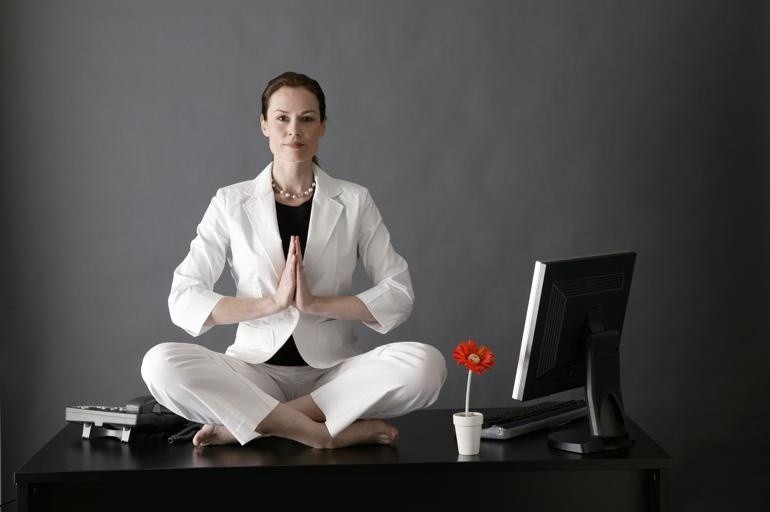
[138,71,448,452]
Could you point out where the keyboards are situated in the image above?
[482,399,588,439]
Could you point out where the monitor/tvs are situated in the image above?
[512,249,637,454]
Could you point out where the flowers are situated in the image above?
[453,339,496,415]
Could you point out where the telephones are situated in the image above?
[64,396,179,442]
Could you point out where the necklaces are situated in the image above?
[271,176,316,200]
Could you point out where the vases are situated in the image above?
[453,412,483,455]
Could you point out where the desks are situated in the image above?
[10,405,674,512]
